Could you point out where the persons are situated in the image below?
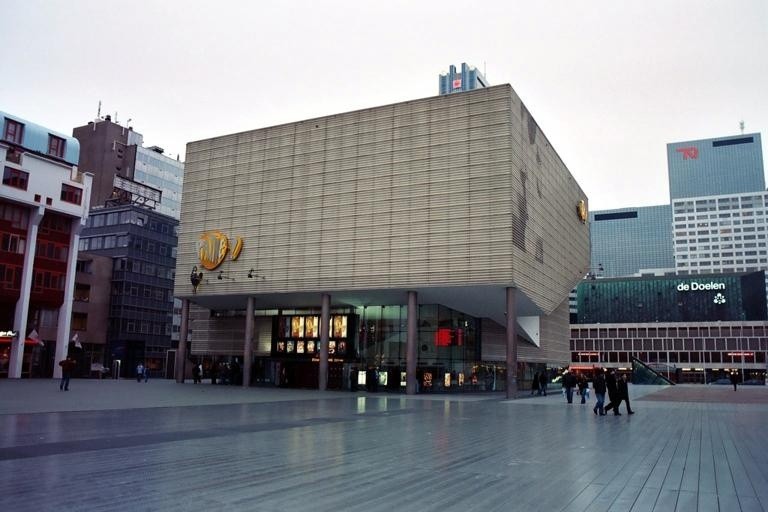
[592,368,606,416]
[613,374,635,415]
[730,372,738,391]
[134,362,145,383]
[602,367,622,417]
[58,355,77,392]
[142,364,150,384]
[191,359,244,386]
[530,369,590,405]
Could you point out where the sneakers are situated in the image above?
[614,411,634,416]
[593,406,608,415]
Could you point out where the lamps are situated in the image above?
[190,266,265,295]
[585,264,604,271]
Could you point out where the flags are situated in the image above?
[72,331,82,350]
[28,328,46,348]
